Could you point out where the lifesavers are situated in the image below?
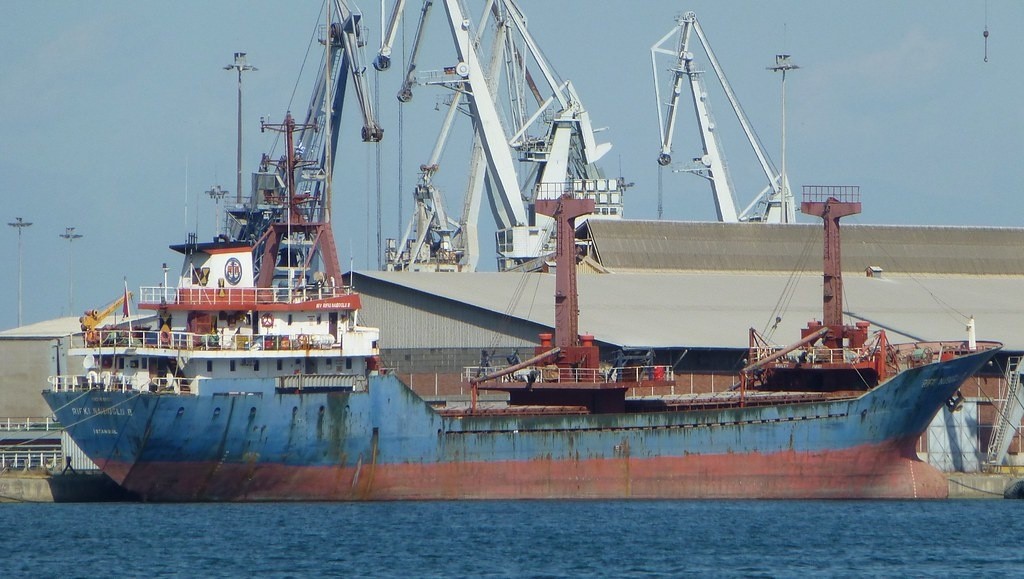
[261,313,274,327]
[298,334,308,345]
[160,331,171,343]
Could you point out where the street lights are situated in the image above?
[765,52,802,224]
[57,226,85,317]
[204,185,230,242]
[219,50,260,205]
[5,217,34,327]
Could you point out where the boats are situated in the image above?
[34,101,1008,506]
